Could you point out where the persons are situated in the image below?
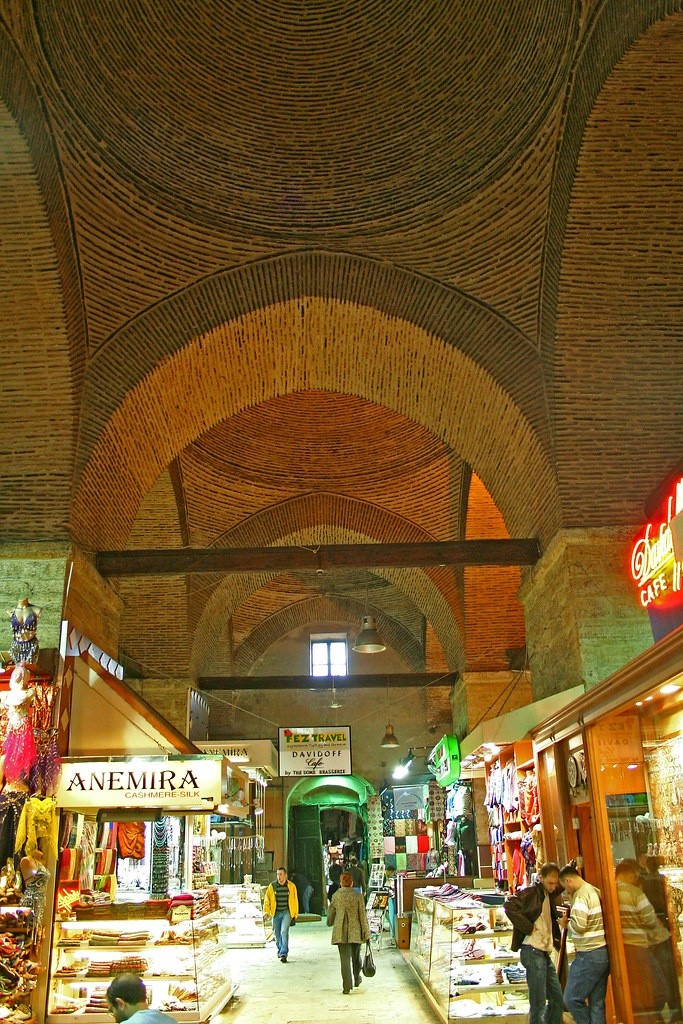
[105,973,178,1024]
[263,867,299,963]
[382,865,395,887]
[6,597,43,663]
[444,821,455,849]
[558,866,610,1024]
[519,771,544,888]
[19,850,52,907]
[459,817,478,875]
[503,858,576,1024]
[4,667,37,730]
[344,858,366,897]
[327,904,328,905]
[615,851,683,1024]
[327,857,343,905]
[327,873,376,994]
[291,869,315,912]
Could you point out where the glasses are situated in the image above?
[107,1005,113,1018]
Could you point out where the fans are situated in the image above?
[316,689,342,709]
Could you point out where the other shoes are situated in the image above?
[355,976,362,987]
[343,990,348,993]
[281,957,286,962]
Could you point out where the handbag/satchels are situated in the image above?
[363,942,376,977]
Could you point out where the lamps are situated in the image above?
[225,788,244,800]
[245,798,263,815]
[352,571,386,653]
[402,747,434,767]
[379,676,399,748]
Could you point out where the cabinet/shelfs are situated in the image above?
[485,741,538,894]
[410,887,532,1024]
[0,902,37,1024]
[216,884,273,948]
[46,907,239,1024]
